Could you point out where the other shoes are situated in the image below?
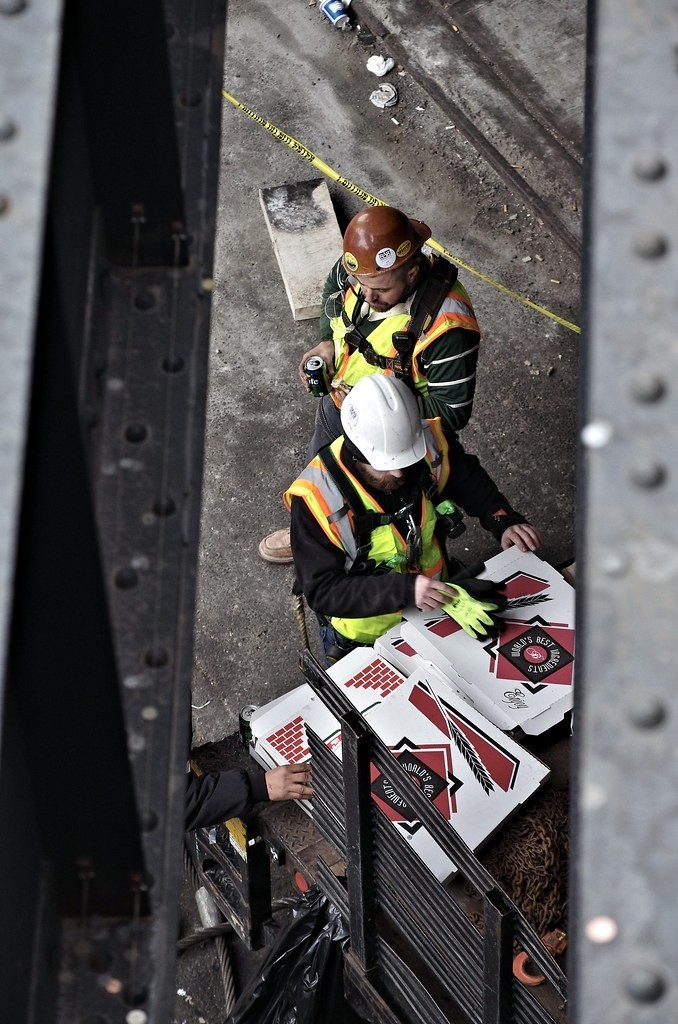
[257,526,294,563]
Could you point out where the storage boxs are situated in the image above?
[247,543,576,882]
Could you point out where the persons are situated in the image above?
[259,206,482,565]
[184,761,315,832]
[282,376,542,671]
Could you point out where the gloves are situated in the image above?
[432,582,507,642]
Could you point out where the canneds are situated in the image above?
[304,356,333,397]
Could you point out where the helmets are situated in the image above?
[340,372,428,472]
[341,204,433,276]
